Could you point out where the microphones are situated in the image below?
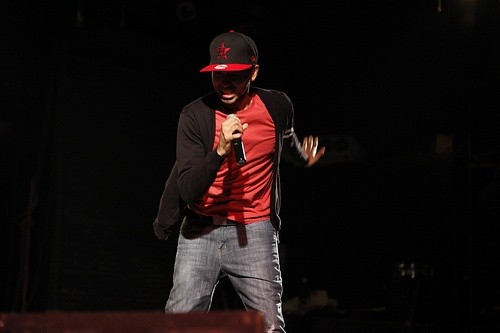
[227,114,246,164]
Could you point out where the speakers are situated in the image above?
[0,311,264,333]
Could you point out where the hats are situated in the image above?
[200,30,258,72]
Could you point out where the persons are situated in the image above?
[153,29,325,332]
